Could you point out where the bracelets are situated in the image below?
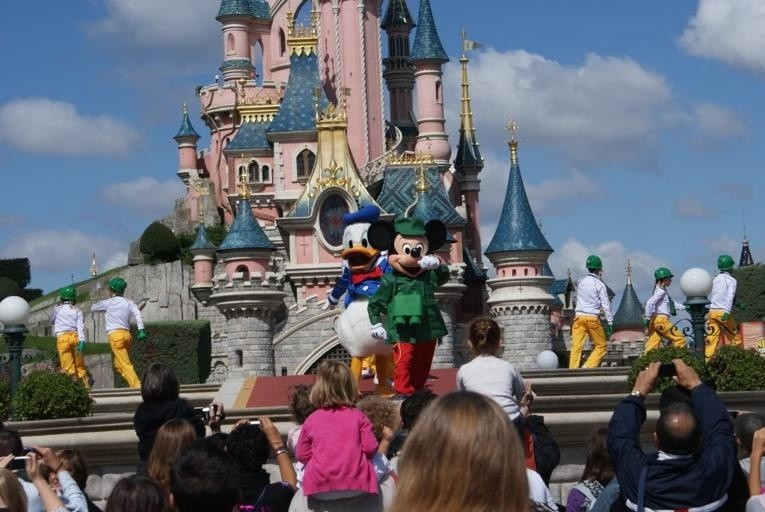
[630,390,645,398]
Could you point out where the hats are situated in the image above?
[343,205,380,224]
[396,217,428,236]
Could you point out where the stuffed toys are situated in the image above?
[320,203,399,398]
[361,215,453,399]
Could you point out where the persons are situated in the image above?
[90,277,149,389]
[1,382,764,511]
[292,359,381,511]
[608,358,738,512]
[48,287,91,389]
[569,255,614,369]
[704,255,742,364]
[132,365,196,468]
[457,318,526,445]
[643,268,687,353]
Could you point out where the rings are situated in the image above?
[644,367,649,369]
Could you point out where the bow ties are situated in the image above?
[350,267,385,286]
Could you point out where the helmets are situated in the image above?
[654,268,674,279]
[587,255,602,269]
[718,254,736,270]
[61,287,78,300]
[110,277,126,293]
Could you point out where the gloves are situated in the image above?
[78,341,85,350]
[136,328,149,339]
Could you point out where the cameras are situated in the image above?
[194,405,215,420]
[659,364,677,377]
[22,447,33,456]
[245,421,261,432]
[8,455,29,470]
